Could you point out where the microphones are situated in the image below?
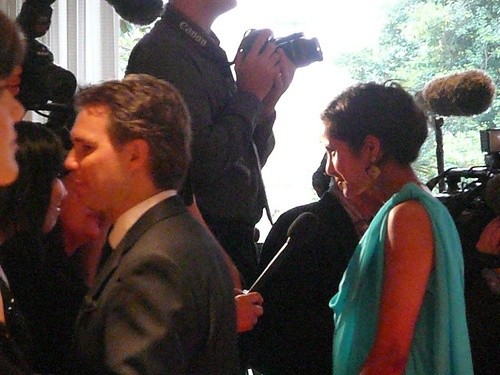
[106,0,163,24]
[414,70,493,117]
[249,212,320,298]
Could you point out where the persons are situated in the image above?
[320,78,474,375]
[0,120,500,375]
[0,11,29,375]
[62,72,242,375]
[123,0,296,293]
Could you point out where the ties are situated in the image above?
[96,224,114,277]
[217,45,274,225]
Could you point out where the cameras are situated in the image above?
[244,29,323,65]
[12,0,77,109]
